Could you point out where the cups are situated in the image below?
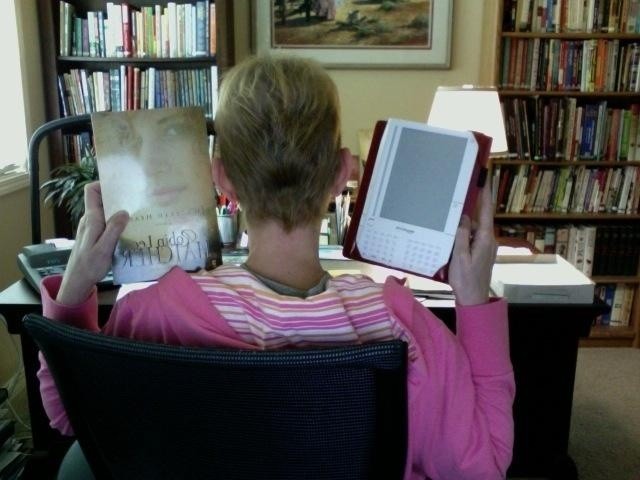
[215,214,239,246]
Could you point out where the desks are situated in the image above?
[0,236,610,479]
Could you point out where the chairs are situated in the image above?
[19,309,412,479]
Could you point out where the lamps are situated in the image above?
[425,84,514,247]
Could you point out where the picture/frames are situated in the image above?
[246,0,455,72]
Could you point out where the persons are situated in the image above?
[37,54,516,480]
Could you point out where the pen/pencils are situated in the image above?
[212,192,238,215]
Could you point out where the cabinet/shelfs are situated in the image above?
[475,0,639,352]
[37,2,236,235]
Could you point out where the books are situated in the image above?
[89,107,224,283]
[58,1,219,169]
